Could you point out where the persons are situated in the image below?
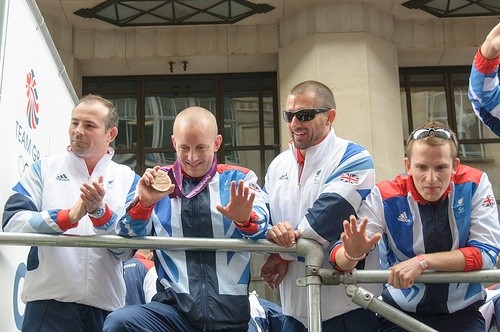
[0,94,142,332]
[467,23,500,137]
[102,106,270,332]
[327,119,500,332]
[259,80,384,332]
[123,248,284,332]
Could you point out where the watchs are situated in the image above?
[88,204,106,219]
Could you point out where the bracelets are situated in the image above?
[344,252,366,260]
[416,254,428,270]
[235,216,250,227]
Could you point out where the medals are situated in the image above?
[151,169,172,192]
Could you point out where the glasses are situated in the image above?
[282,108,328,123]
[406,128,458,151]
